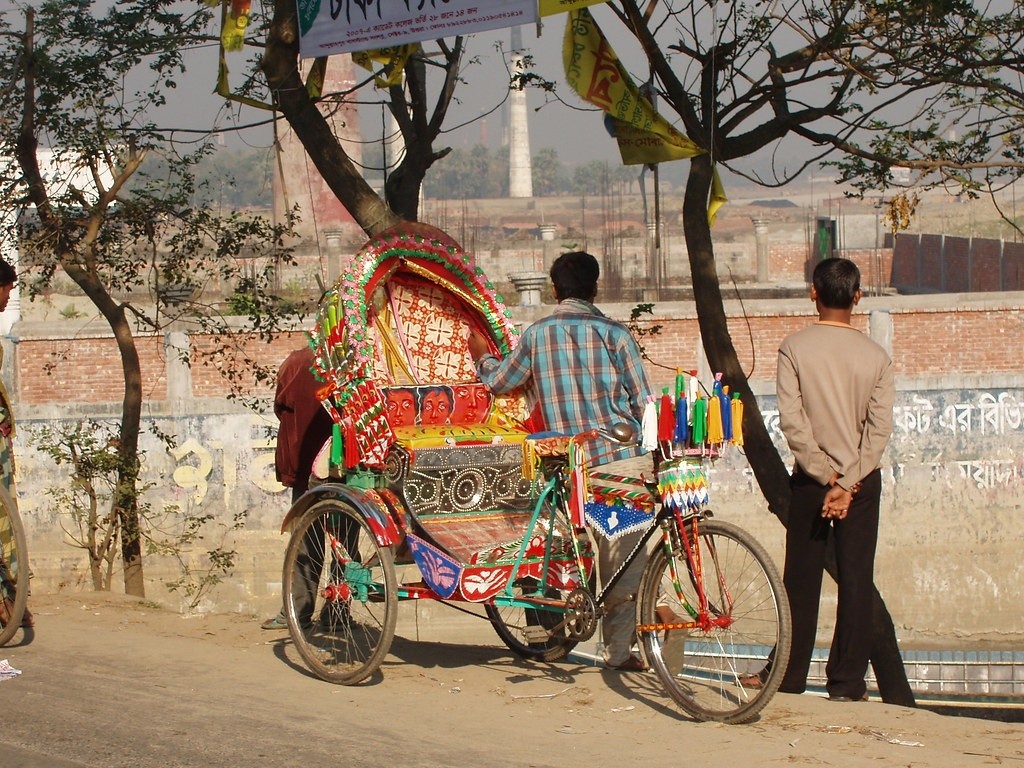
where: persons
[262,346,360,630]
[734,258,894,700]
[0,256,35,625]
[467,251,653,674]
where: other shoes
[0,597,34,627]
[320,621,356,632]
[829,691,868,701]
[604,654,645,671]
[734,670,780,689]
[661,618,687,677]
[262,613,313,629]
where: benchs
[376,382,548,517]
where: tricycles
[274,218,793,730]
